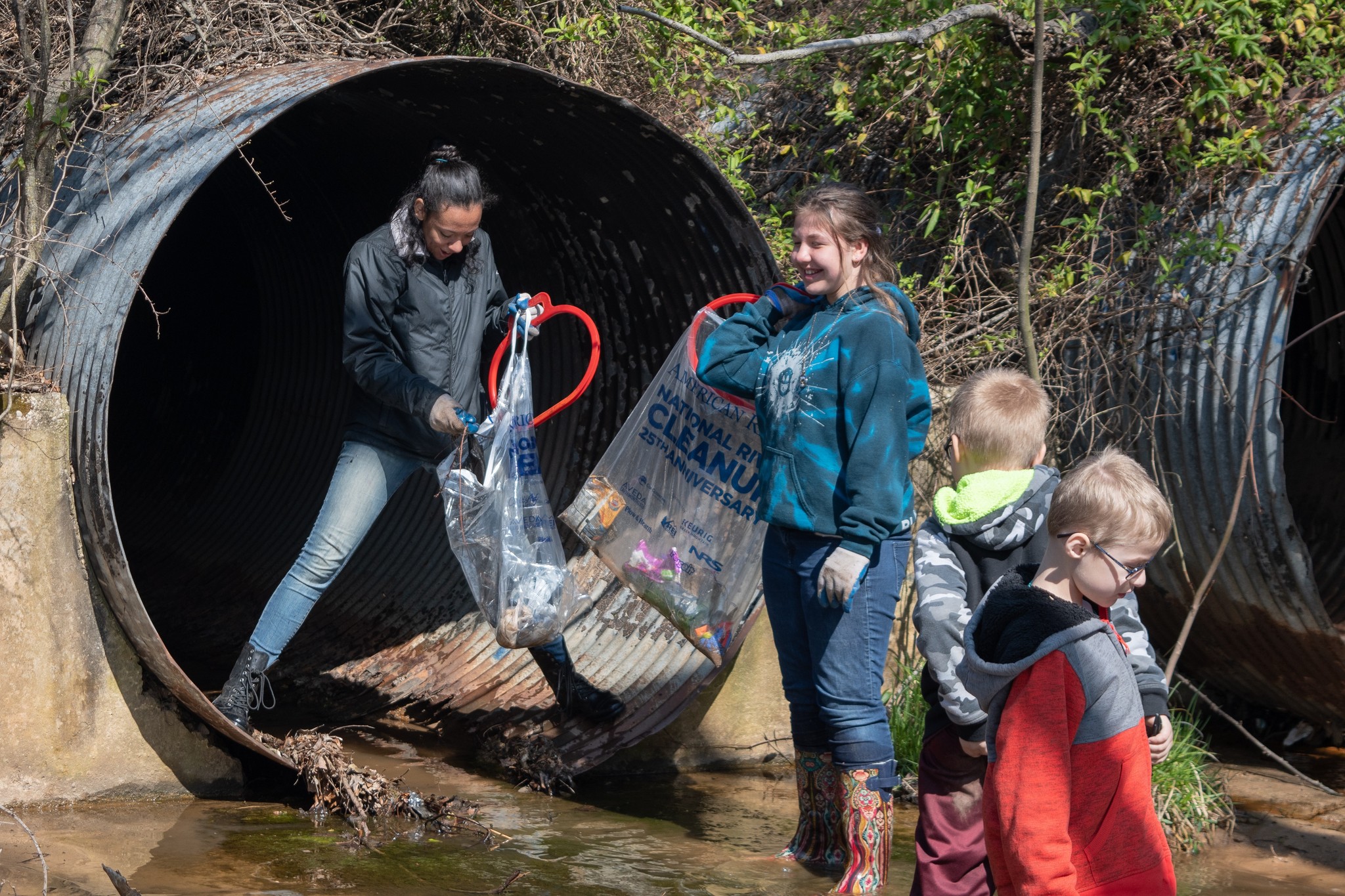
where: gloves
[429,393,480,435]
[508,291,545,340]
[816,546,873,614]
[767,279,825,318]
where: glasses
[1055,530,1154,581]
[943,432,958,460]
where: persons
[914,369,1175,896]
[694,184,932,896]
[215,145,625,733]
[958,444,1184,896]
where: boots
[532,634,625,722]
[212,640,281,731]
[828,757,900,896]
[770,748,846,865]
